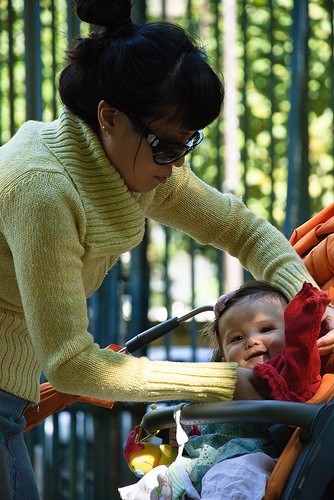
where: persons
[0,1,334,499]
[119,281,332,500]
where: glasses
[120,106,204,166]
